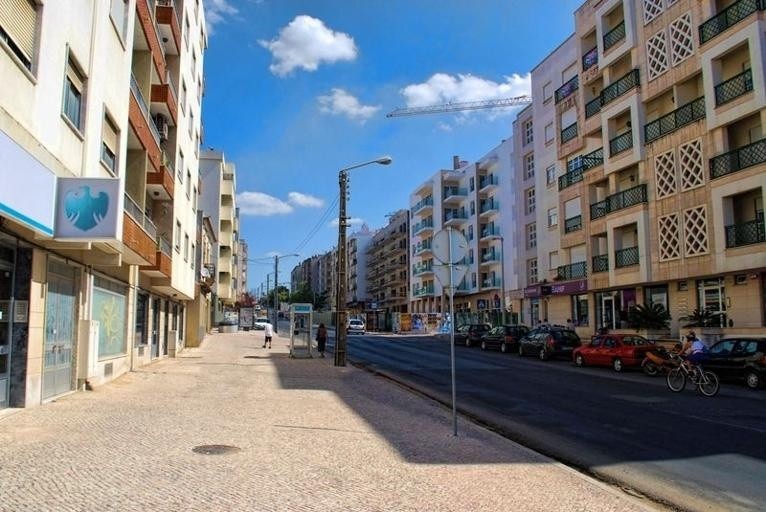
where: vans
[258,310,268,317]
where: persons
[543,319,550,326]
[566,317,576,332]
[262,318,274,349]
[536,319,543,330]
[315,323,329,357]
[678,329,710,384]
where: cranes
[386,95,531,118]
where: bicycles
[666,353,719,397]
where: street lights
[334,156,392,366]
[267,254,299,333]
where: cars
[255,305,261,312]
[347,319,365,335]
[254,316,267,329]
